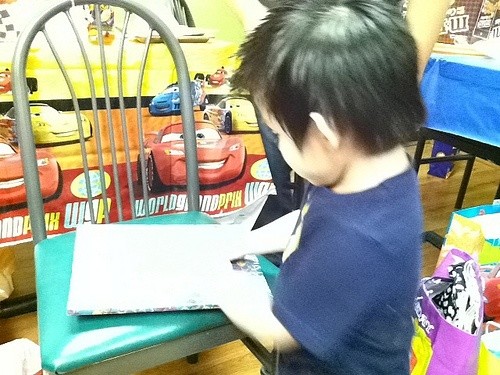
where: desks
[413,54,500,210]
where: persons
[172,0,451,375]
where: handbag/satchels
[478,321,500,375]
[408,248,484,375]
[435,199,500,271]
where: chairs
[11,0,280,375]
